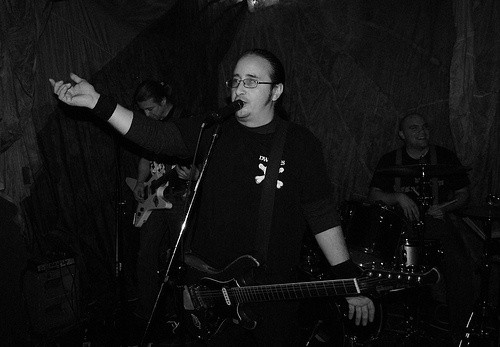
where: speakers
[24,266,81,336]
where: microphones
[203,100,243,127]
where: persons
[48,49,375,325]
[370,113,470,221]
[132,79,199,285]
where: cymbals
[454,205,500,221]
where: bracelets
[330,257,355,278]
[91,91,116,120]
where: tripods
[456,203,500,347]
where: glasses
[223,79,274,88]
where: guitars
[125,165,189,229]
[159,252,440,345]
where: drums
[366,201,415,266]
[341,199,367,235]
